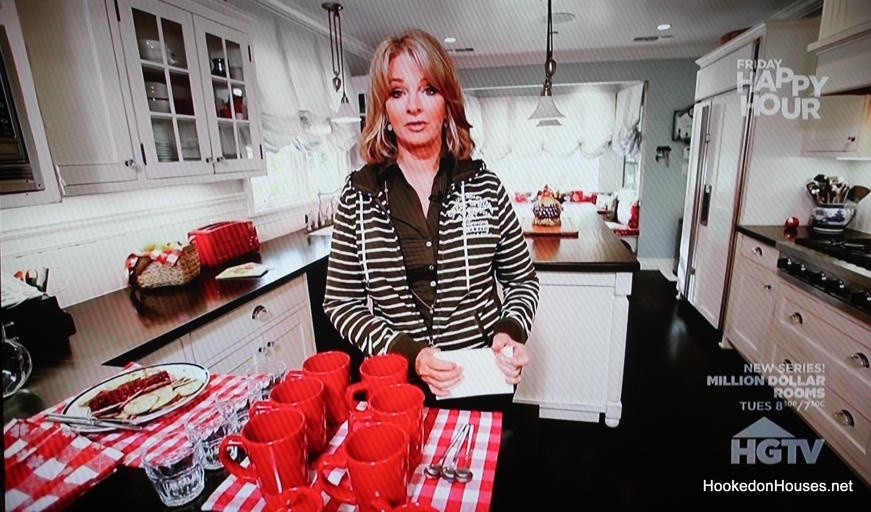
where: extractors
[808,2,871,98]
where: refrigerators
[670,16,806,334]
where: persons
[320,27,540,410]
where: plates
[155,139,174,160]
[55,361,212,436]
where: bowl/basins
[145,81,174,112]
[139,38,169,61]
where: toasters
[185,218,263,266]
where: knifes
[43,411,148,437]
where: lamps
[527,0,564,127]
[323,2,361,122]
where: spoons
[427,422,477,490]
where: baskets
[135,243,202,288]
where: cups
[213,57,231,75]
[142,349,426,511]
[218,91,246,119]
[809,204,858,233]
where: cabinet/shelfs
[717,234,775,381]
[105,0,269,187]
[138,274,317,386]
[9,0,139,197]
[758,279,868,499]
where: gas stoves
[775,236,871,326]
